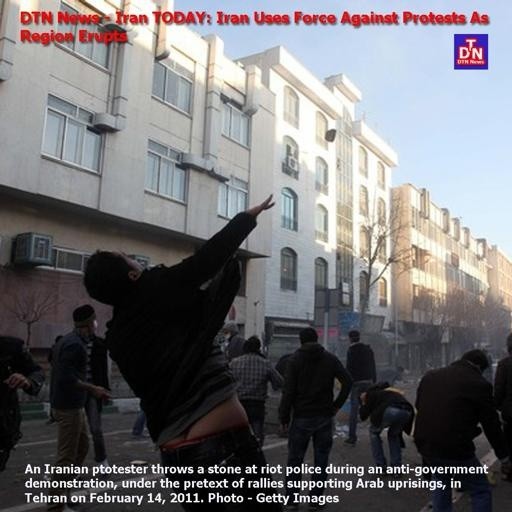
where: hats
[300,327,318,344]
[73,305,97,327]
[462,349,488,370]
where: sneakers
[48,459,110,511]
[376,464,402,474]
[344,438,356,443]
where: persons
[0,305,512,512]
[81,192,306,512]
